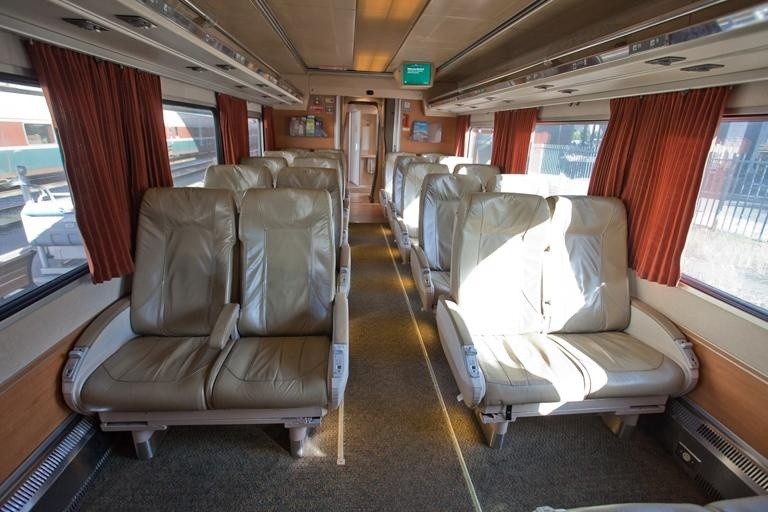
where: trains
[1,81,261,232]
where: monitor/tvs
[399,61,435,90]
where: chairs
[435,192,700,446]
[63,188,349,464]
[530,490,768,512]
[380,155,573,313]
[204,147,352,295]
